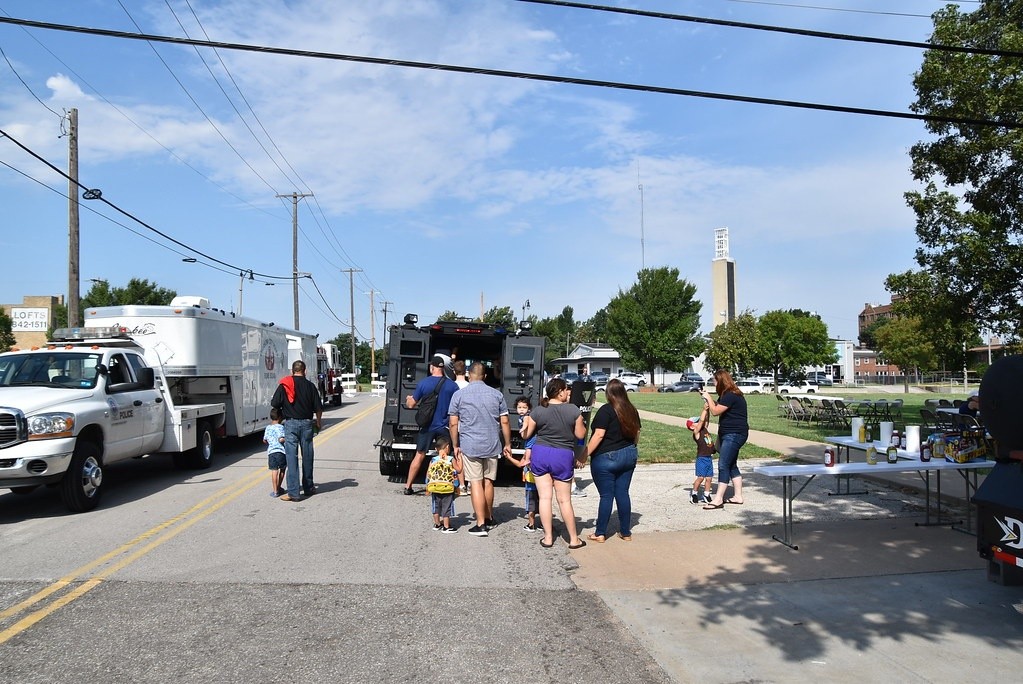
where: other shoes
[280,495,300,502]
[404,488,414,495]
[270,487,284,498]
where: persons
[263,408,287,498]
[513,396,533,434]
[575,379,641,543]
[434,340,502,495]
[686,402,716,504]
[271,360,322,501]
[447,363,512,536]
[784,371,802,388]
[700,371,749,510]
[526,378,586,550]
[425,437,463,534]
[578,367,593,382]
[959,388,979,418]
[404,356,460,496]
[504,434,547,532]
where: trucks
[0,295,320,516]
[373,312,548,477]
[316,344,343,408]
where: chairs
[919,398,979,436]
[776,394,905,433]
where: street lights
[522,299,531,321]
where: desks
[936,407,981,433]
[783,395,844,420]
[840,399,901,429]
[824,436,997,527]
[753,457,996,550]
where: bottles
[824,446,835,467]
[859,425,866,443]
[865,425,873,443]
[866,444,877,465]
[901,432,906,450]
[920,441,931,462]
[891,430,901,448]
[886,444,898,464]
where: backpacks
[426,452,454,494]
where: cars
[733,380,766,395]
[707,372,833,387]
[657,382,700,393]
[588,372,609,386]
[777,380,820,393]
[615,372,647,387]
[680,373,706,386]
[552,372,579,389]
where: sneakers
[537,524,544,531]
[441,527,457,533]
[432,523,444,531]
[702,490,713,503]
[689,489,698,505]
[484,516,498,530]
[523,523,536,533]
[458,485,472,496]
[467,524,488,537]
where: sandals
[587,534,605,543]
[617,532,631,541]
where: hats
[427,356,444,368]
[686,416,700,427]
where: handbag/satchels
[414,376,447,433]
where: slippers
[540,537,552,547]
[724,498,743,504]
[703,503,724,510]
[568,538,586,549]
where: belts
[285,418,299,420]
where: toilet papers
[880,421,893,446]
[905,425,921,453]
[852,418,864,441]
